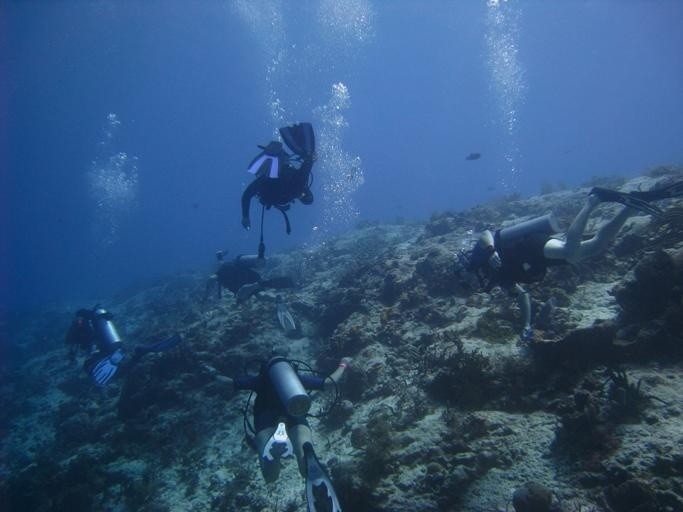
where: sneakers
[265,142,283,154]
[135,346,152,355]
[276,294,282,304]
[303,442,322,481]
[631,191,649,202]
[588,187,620,202]
[257,280,271,288]
[111,347,126,363]
[273,422,288,440]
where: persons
[203,248,283,303]
[202,356,353,483]
[241,152,313,232]
[69,309,153,367]
[453,193,640,340]
